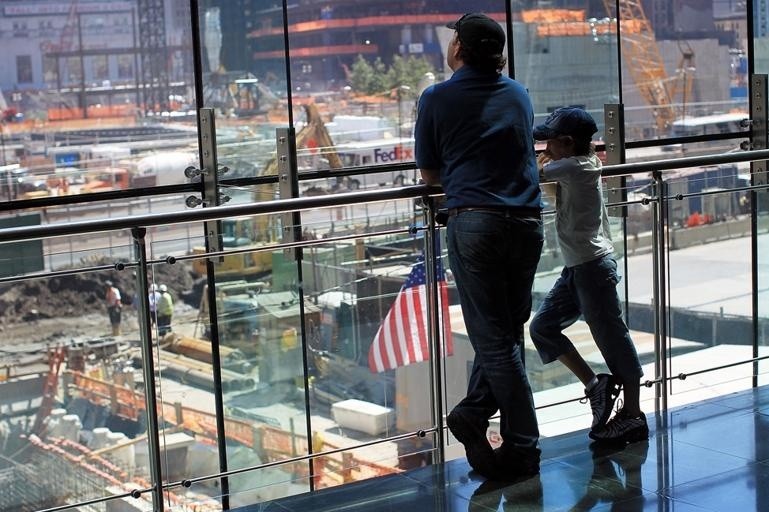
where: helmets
[104,280,168,292]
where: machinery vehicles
[265,96,333,127]
[182,98,350,300]
[81,152,201,194]
[202,278,265,335]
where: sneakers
[588,398,648,441]
[446,412,540,470]
[580,373,622,431]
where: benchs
[330,399,395,437]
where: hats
[533,107,597,141]
[445,12,505,54]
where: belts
[435,207,507,226]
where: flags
[367,226,454,374]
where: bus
[46,80,187,122]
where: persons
[529,105,650,444]
[415,12,543,475]
[567,440,646,512]
[467,472,544,512]
[102,279,173,337]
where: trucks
[0,163,47,198]
[661,114,748,156]
[310,264,459,367]
[336,138,416,189]
[627,165,750,221]
